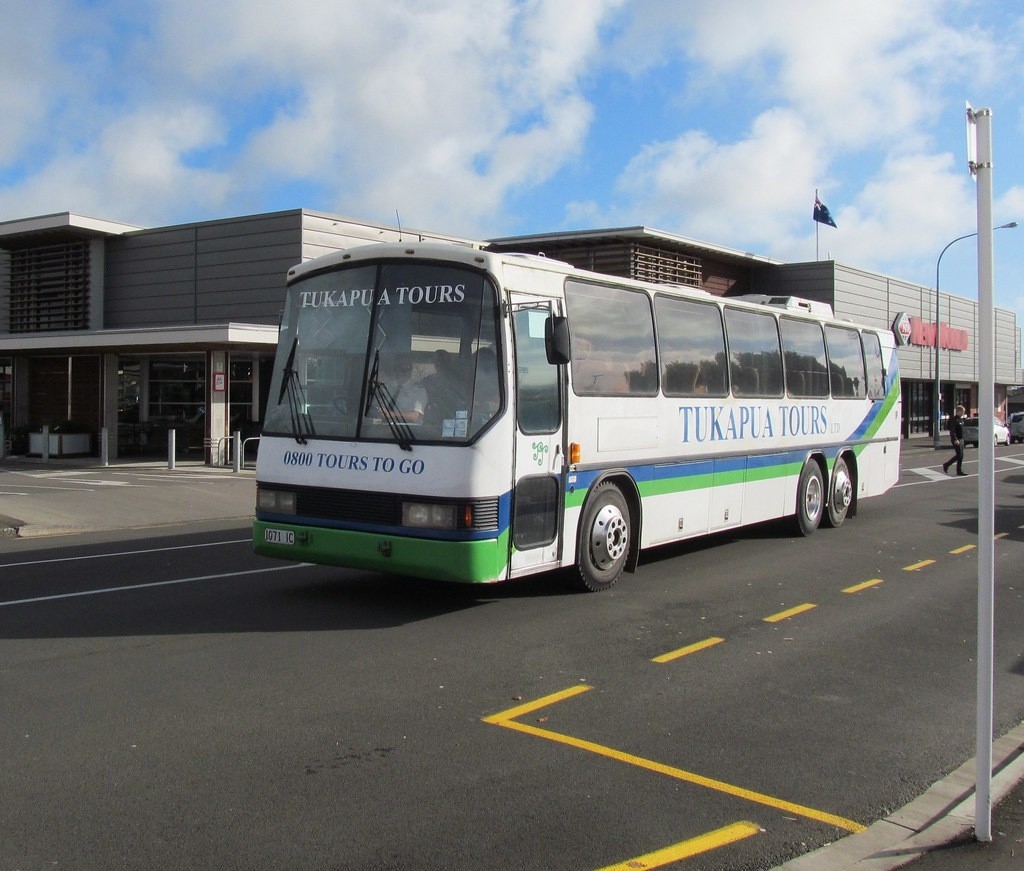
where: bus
[251,234,903,593]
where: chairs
[680,363,702,391]
[741,366,760,394]
[790,370,805,397]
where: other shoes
[943,464,948,473]
[957,472,968,476]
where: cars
[961,415,1012,447]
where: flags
[813,199,836,228]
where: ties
[387,385,401,410]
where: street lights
[933,222,1019,448]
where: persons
[367,354,427,424]
[469,347,501,403]
[943,406,968,475]
[422,348,461,403]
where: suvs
[1008,412,1024,442]
